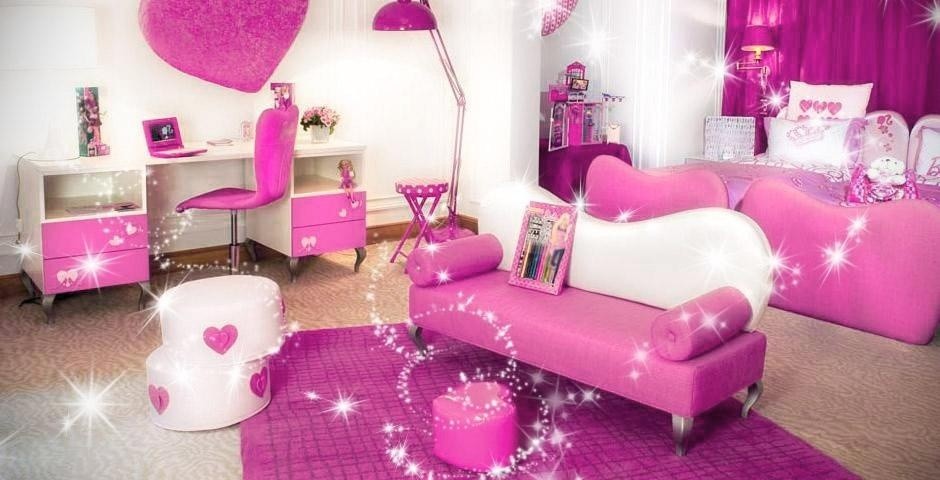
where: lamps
[372,0,467,240]
[735,24,774,77]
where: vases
[311,125,329,143]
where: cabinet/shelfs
[16,138,366,324]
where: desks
[540,138,632,204]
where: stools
[146,344,273,431]
[156,274,287,363]
[389,177,449,273]
[428,381,520,472]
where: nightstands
[685,153,715,164]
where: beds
[584,81,939,344]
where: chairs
[176,104,299,274]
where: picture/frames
[507,201,576,296]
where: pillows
[861,109,911,172]
[789,80,873,119]
[907,113,939,186]
[761,116,852,171]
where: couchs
[405,176,777,457]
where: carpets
[241,320,863,478]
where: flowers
[300,103,340,135]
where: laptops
[142,117,207,158]
